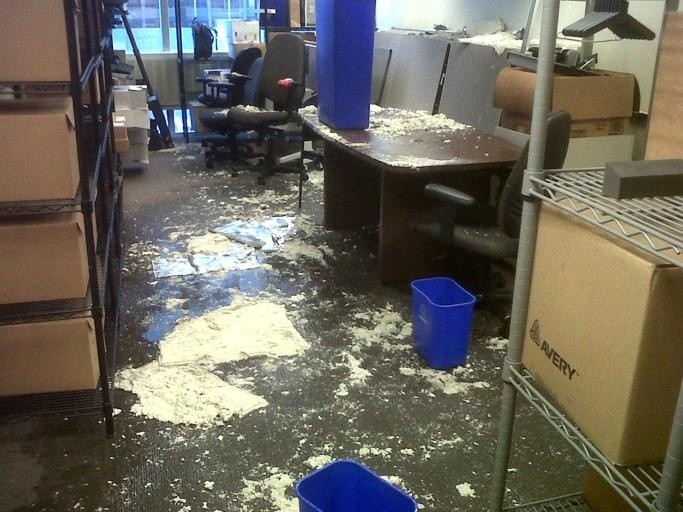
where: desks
[294,104,523,290]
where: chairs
[407,112,577,340]
[194,31,313,188]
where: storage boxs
[3,319,102,393]
[3,2,86,86]
[0,217,90,310]
[110,79,153,167]
[522,193,682,470]
[1,97,86,205]
[490,65,645,140]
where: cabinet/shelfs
[487,0,683,512]
[0,0,131,440]
